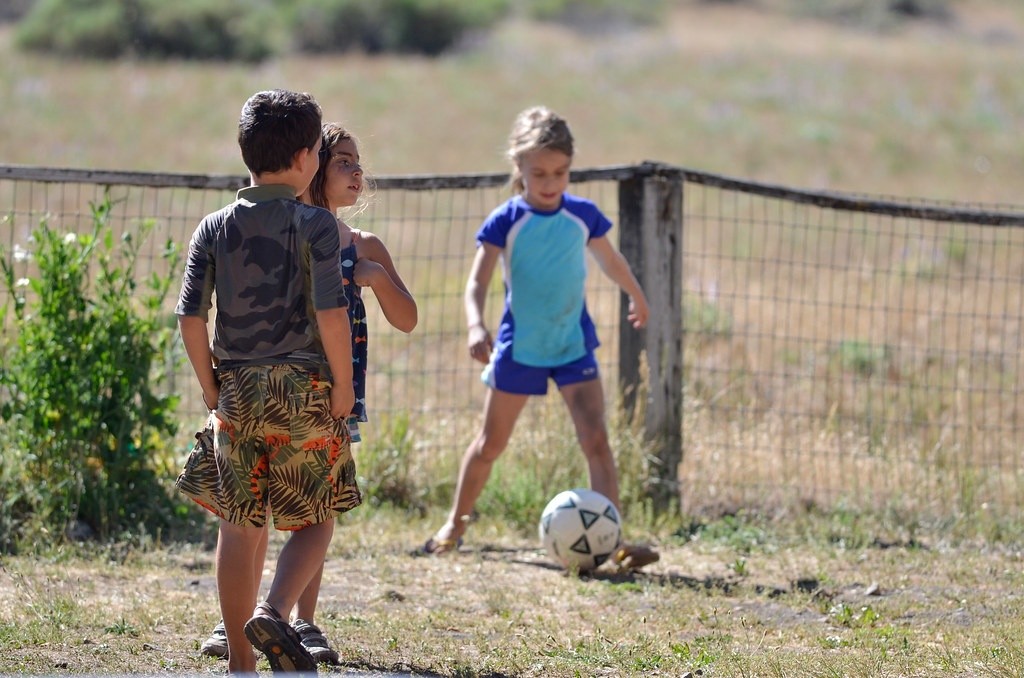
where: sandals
[245,602,319,678]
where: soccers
[538,486,624,571]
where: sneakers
[201,616,229,657]
[287,618,338,662]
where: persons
[424,106,660,568]
[198,121,418,662]
[174,90,362,678]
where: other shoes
[410,536,464,560]
[613,540,660,570]
